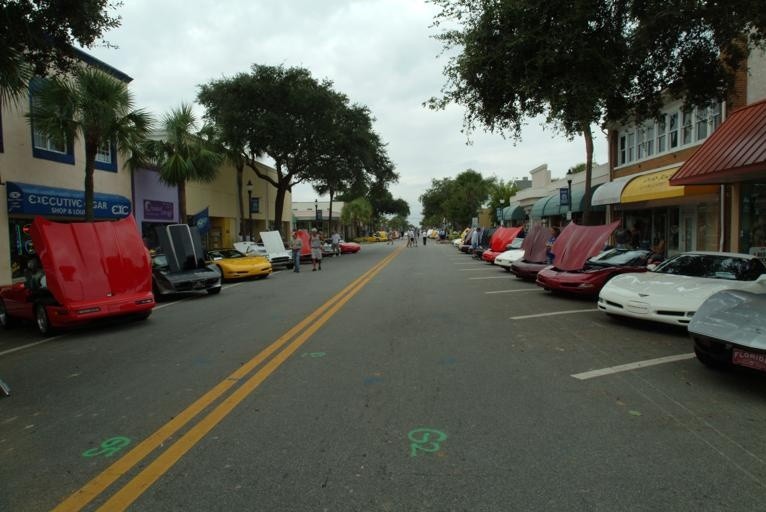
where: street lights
[565,168,575,221]
[314,198,319,227]
[499,197,506,227]
[245,177,255,242]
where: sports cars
[233,230,390,272]
[428,223,558,281]
[590,249,766,334]
[682,284,766,378]
[535,220,665,305]
[204,246,275,285]
[146,221,227,304]
[0,212,159,339]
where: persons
[545,226,561,266]
[284,227,341,273]
[470,228,480,258]
[387,229,427,248]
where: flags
[192,208,211,235]
